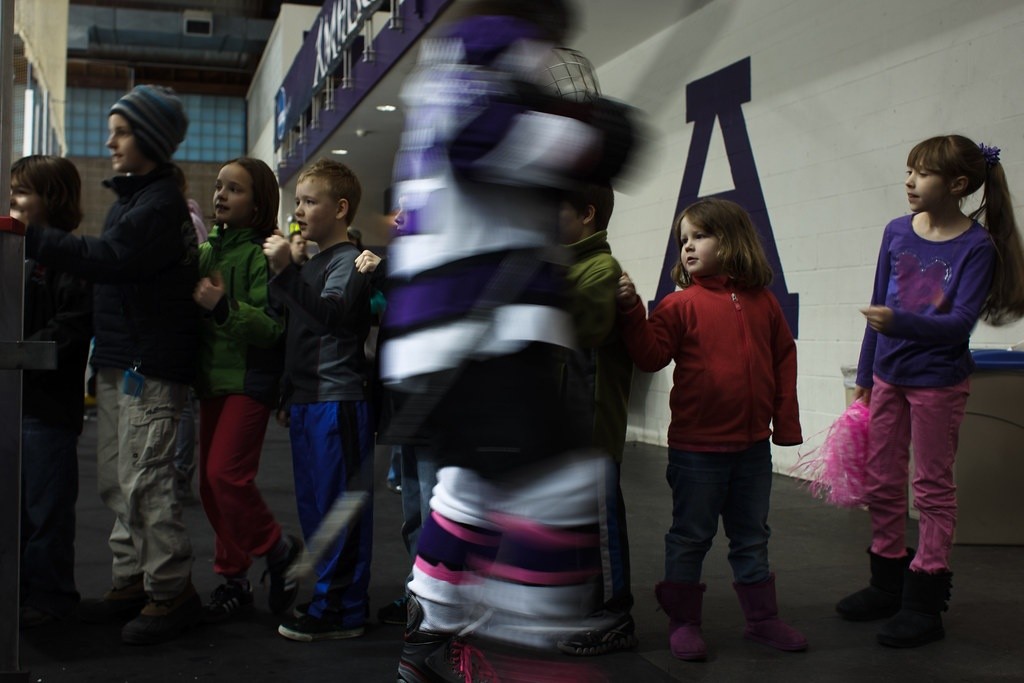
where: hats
[107,84,189,167]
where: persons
[287,230,311,263]
[353,0,637,683]
[262,158,372,639]
[9,84,199,646]
[553,182,634,655]
[196,158,305,622]
[11,155,94,628]
[162,163,207,244]
[620,198,808,661]
[837,135,1024,650]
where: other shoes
[20,589,83,625]
[387,480,401,492]
[78,587,148,623]
[122,583,206,647]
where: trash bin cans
[841,365,858,409]
[908,348,1024,548]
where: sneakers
[557,610,641,657]
[293,601,312,616]
[377,596,408,626]
[264,534,306,618]
[274,611,367,641]
[201,577,259,628]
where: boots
[732,572,810,654]
[834,545,914,623]
[875,568,953,647]
[653,575,711,662]
